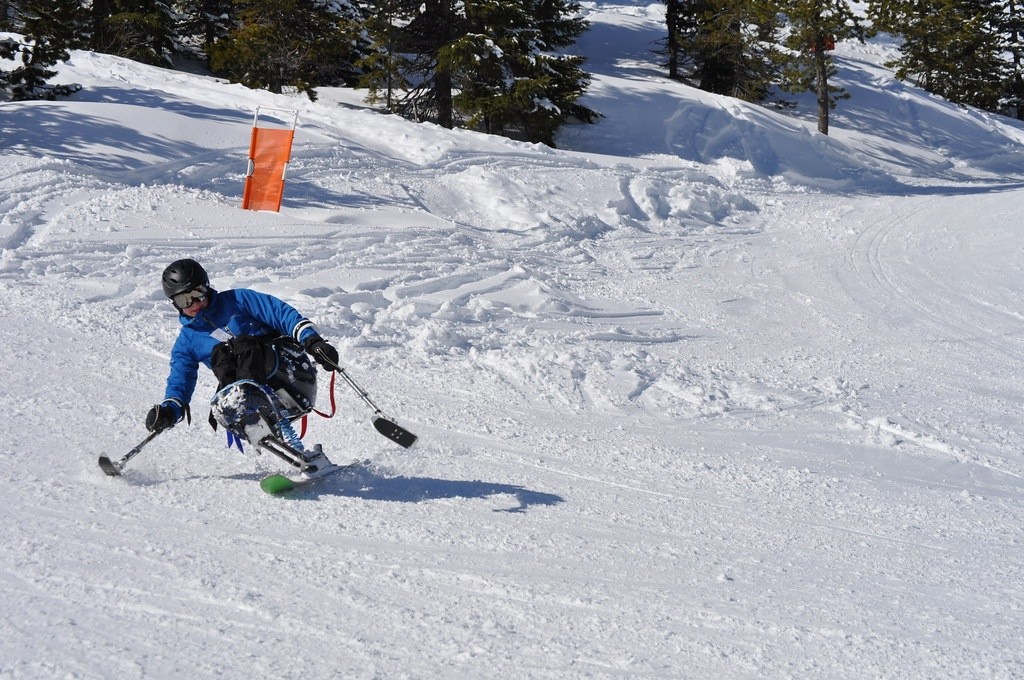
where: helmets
[161,259,208,299]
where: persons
[145,258,340,432]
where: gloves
[305,335,339,372]
[146,406,176,432]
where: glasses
[173,284,209,309]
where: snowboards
[259,457,363,495]
